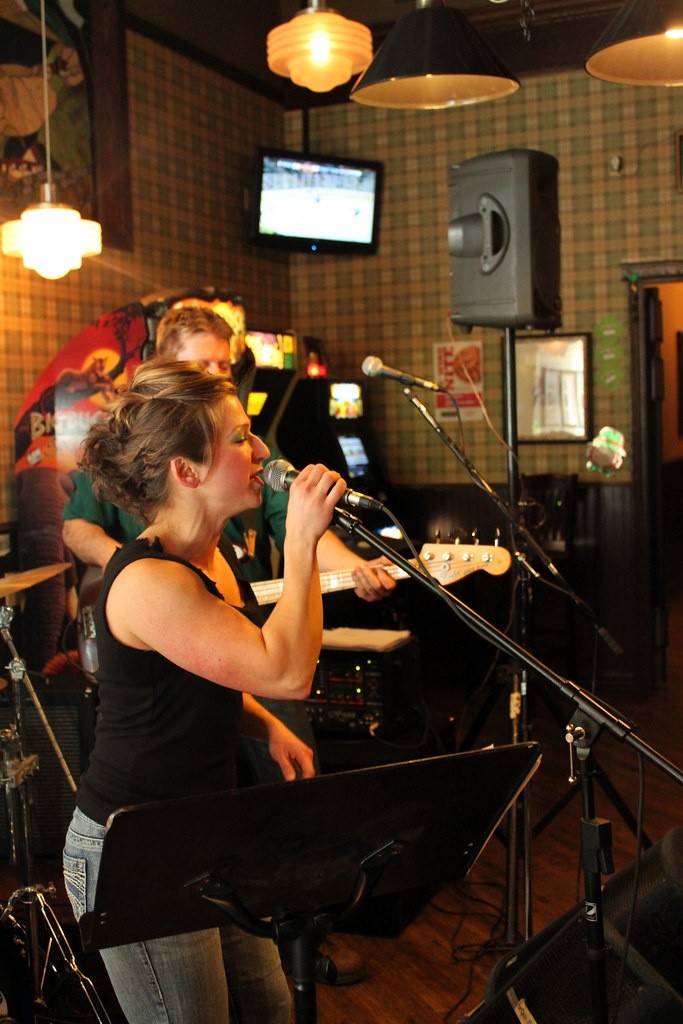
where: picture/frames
[504,331,592,444]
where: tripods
[0,605,112,1024]
[457,328,655,850]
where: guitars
[251,526,511,608]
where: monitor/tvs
[251,145,384,256]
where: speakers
[446,147,562,335]
[0,677,100,856]
[458,824,683,1024]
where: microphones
[361,356,447,394]
[261,459,385,515]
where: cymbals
[1,560,71,598]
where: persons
[62,353,347,1024]
[62,304,396,981]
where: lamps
[586,0,682,86]
[0,0,102,281]
[351,0,521,108]
[265,0,376,93]
